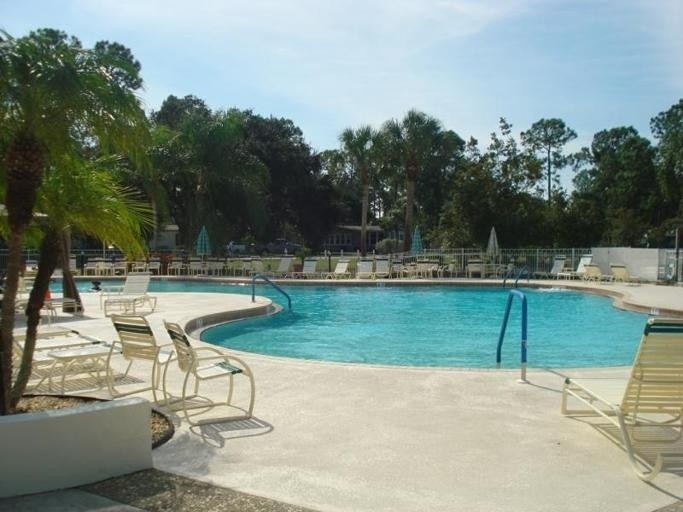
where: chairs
[13,273,255,427]
[83,253,640,285]
[561,317,683,482]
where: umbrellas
[0,205,53,227]
[61,225,84,312]
[411,224,424,254]
[485,226,500,257]
[196,225,212,256]
